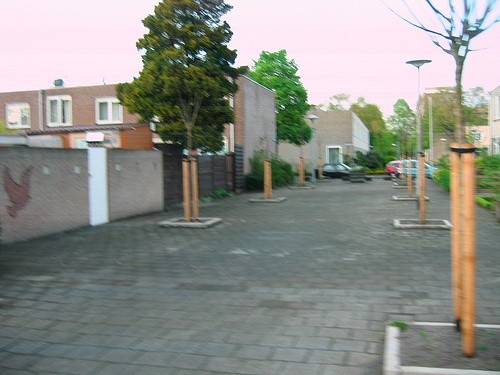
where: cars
[403,160,439,179]
[313,162,371,180]
[384,160,404,178]
[304,114,320,182]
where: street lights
[406,59,432,210]
